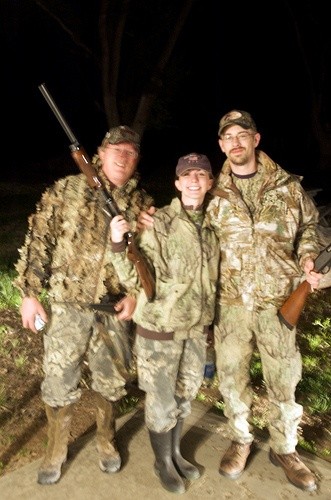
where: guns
[276,245,331,332]
[37,82,155,304]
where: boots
[172,417,199,480]
[147,426,185,494]
[37,404,74,483]
[92,390,122,473]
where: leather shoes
[219,440,255,479]
[269,447,315,491]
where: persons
[135,111,331,490]
[110,155,221,494]
[12,125,153,484]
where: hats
[102,126,141,152]
[218,110,252,136]
[175,153,211,176]
